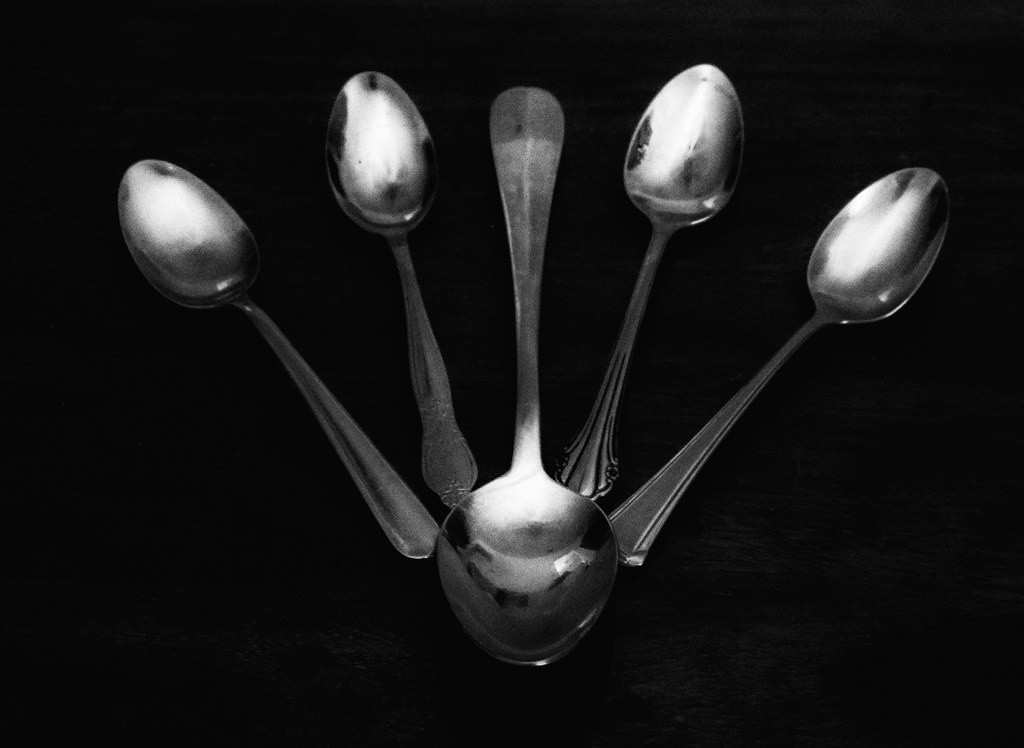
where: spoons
[607,167,952,566]
[556,63,745,494]
[322,70,481,509]
[435,88,617,667]
[119,159,440,559]
